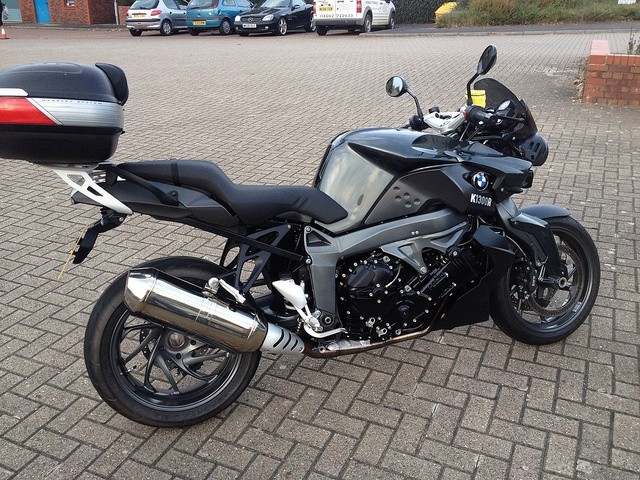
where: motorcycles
[0,45,600,428]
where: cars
[233,0,316,35]
[125,0,188,36]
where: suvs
[185,0,253,35]
[313,0,395,34]
